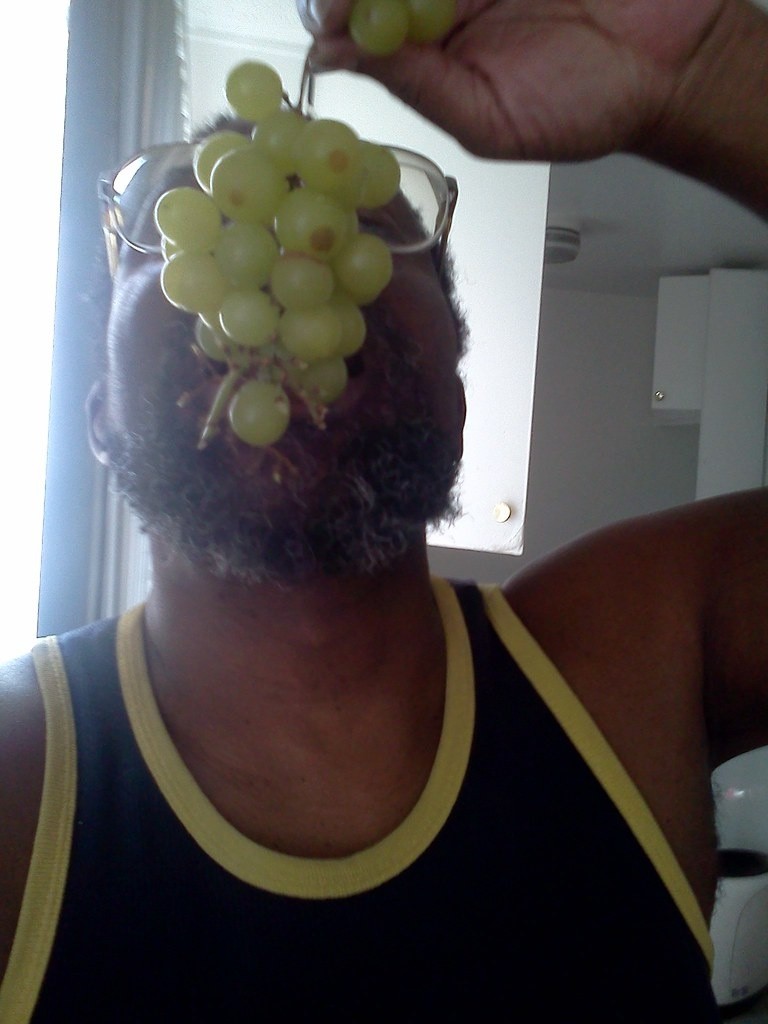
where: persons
[0,0,768,1024]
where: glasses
[97,139,458,279]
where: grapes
[150,1,459,448]
